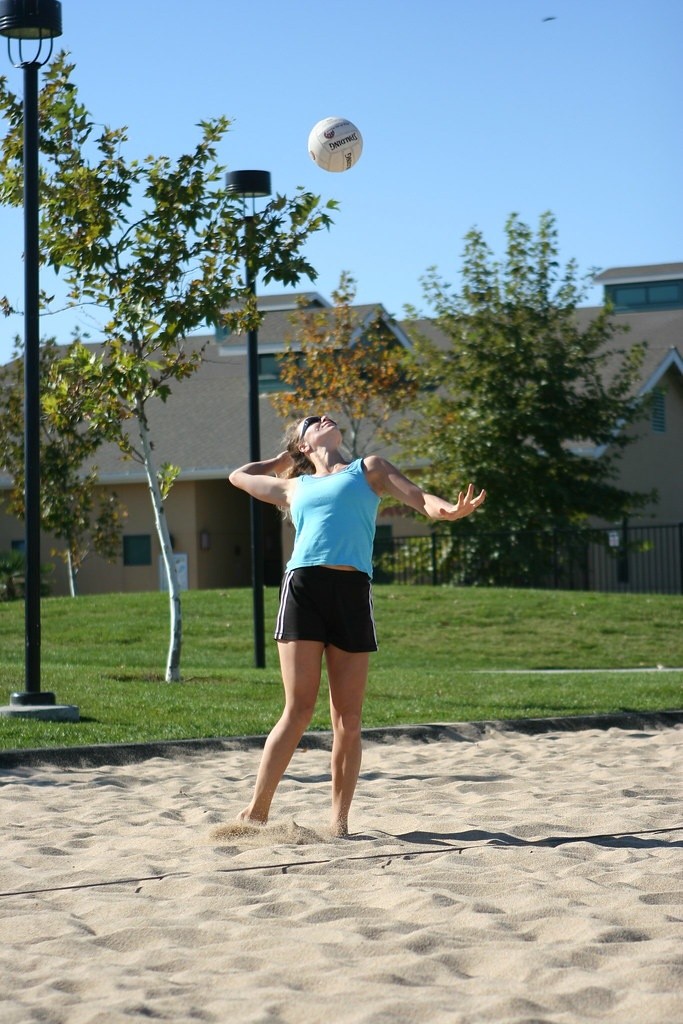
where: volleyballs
[306,115,365,174]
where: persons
[228,414,487,835]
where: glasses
[300,417,336,439]
